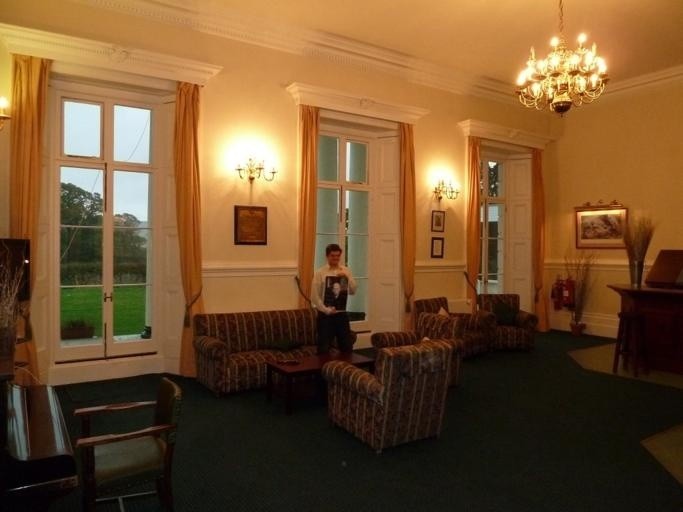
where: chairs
[320,339,453,454]
[477,293,539,351]
[72,375,183,512]
[413,296,491,356]
[369,311,465,386]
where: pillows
[438,307,450,318]
[491,301,517,326]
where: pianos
[1,327,79,511]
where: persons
[325,282,348,310]
[310,243,358,355]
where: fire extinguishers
[551,274,575,311]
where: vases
[628,259,645,287]
[568,322,585,338]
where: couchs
[190,307,357,392]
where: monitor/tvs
[0,238,30,302]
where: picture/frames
[574,205,629,249]
[231,204,268,246]
[430,236,443,258]
[430,210,445,233]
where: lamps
[433,179,460,203]
[513,1,610,116]
[0,96,13,131]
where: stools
[611,309,650,378]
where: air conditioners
[236,158,277,186]
